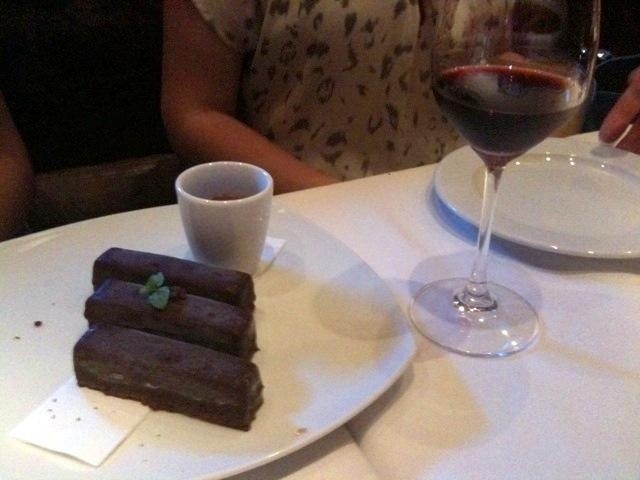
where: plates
[1,199,420,480]
[432,135,640,259]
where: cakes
[85,280,259,362]
[92,248,255,311]
[73,328,264,434]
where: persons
[162,0,583,194]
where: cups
[175,160,276,277]
[409,0,603,364]
